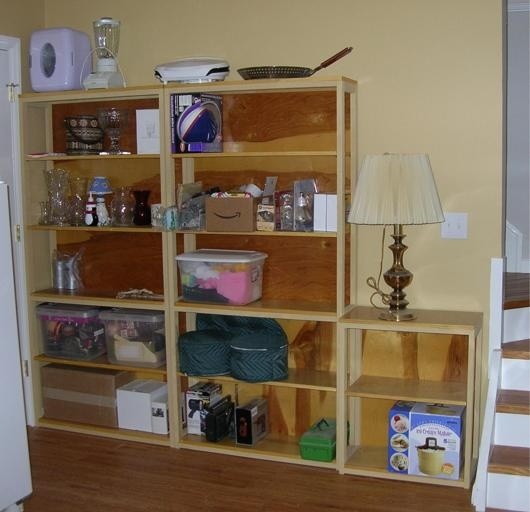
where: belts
[95,107,131,155]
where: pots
[237,45,354,80]
[416,436,446,476]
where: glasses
[346,152,446,322]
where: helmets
[390,413,409,471]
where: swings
[80,14,127,90]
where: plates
[335,303,485,490]
[18,75,359,470]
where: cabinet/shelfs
[35,169,151,227]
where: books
[298,415,350,461]
[387,400,466,481]
[34,301,169,435]
[177,313,291,384]
[173,247,269,307]
[203,190,274,233]
